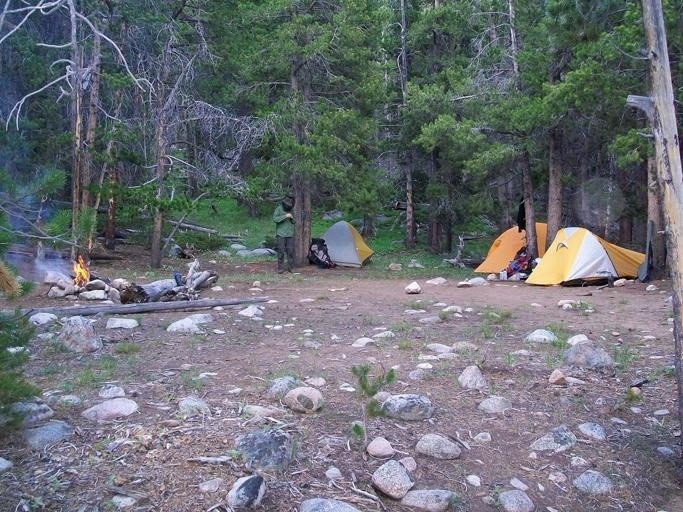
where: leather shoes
[278,269,284,274]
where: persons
[272,194,297,274]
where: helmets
[282,192,295,212]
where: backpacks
[308,238,331,269]
[501,246,533,280]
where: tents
[319,221,376,269]
[473,221,547,274]
[524,227,645,287]
